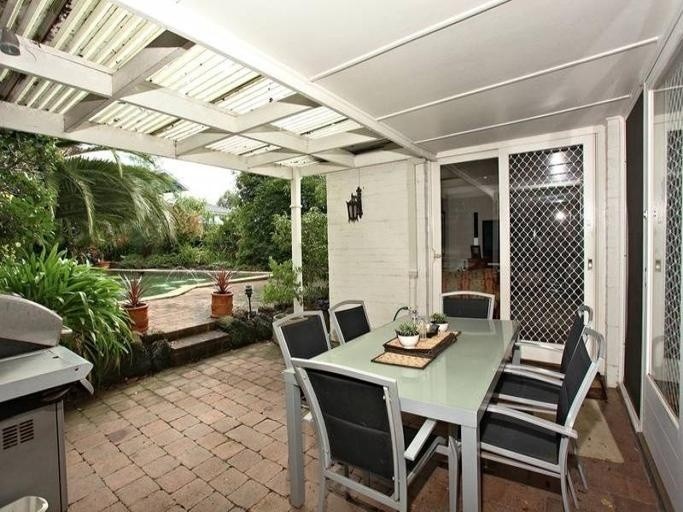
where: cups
[396,334,420,348]
[429,321,448,332]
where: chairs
[441,290,496,319]
[447,327,604,512]
[289,356,446,512]
[328,299,372,345]
[273,311,330,411]
[496,305,594,491]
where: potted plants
[122,270,151,334]
[428,313,448,332]
[395,320,421,348]
[206,261,240,318]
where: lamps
[346,187,363,224]
